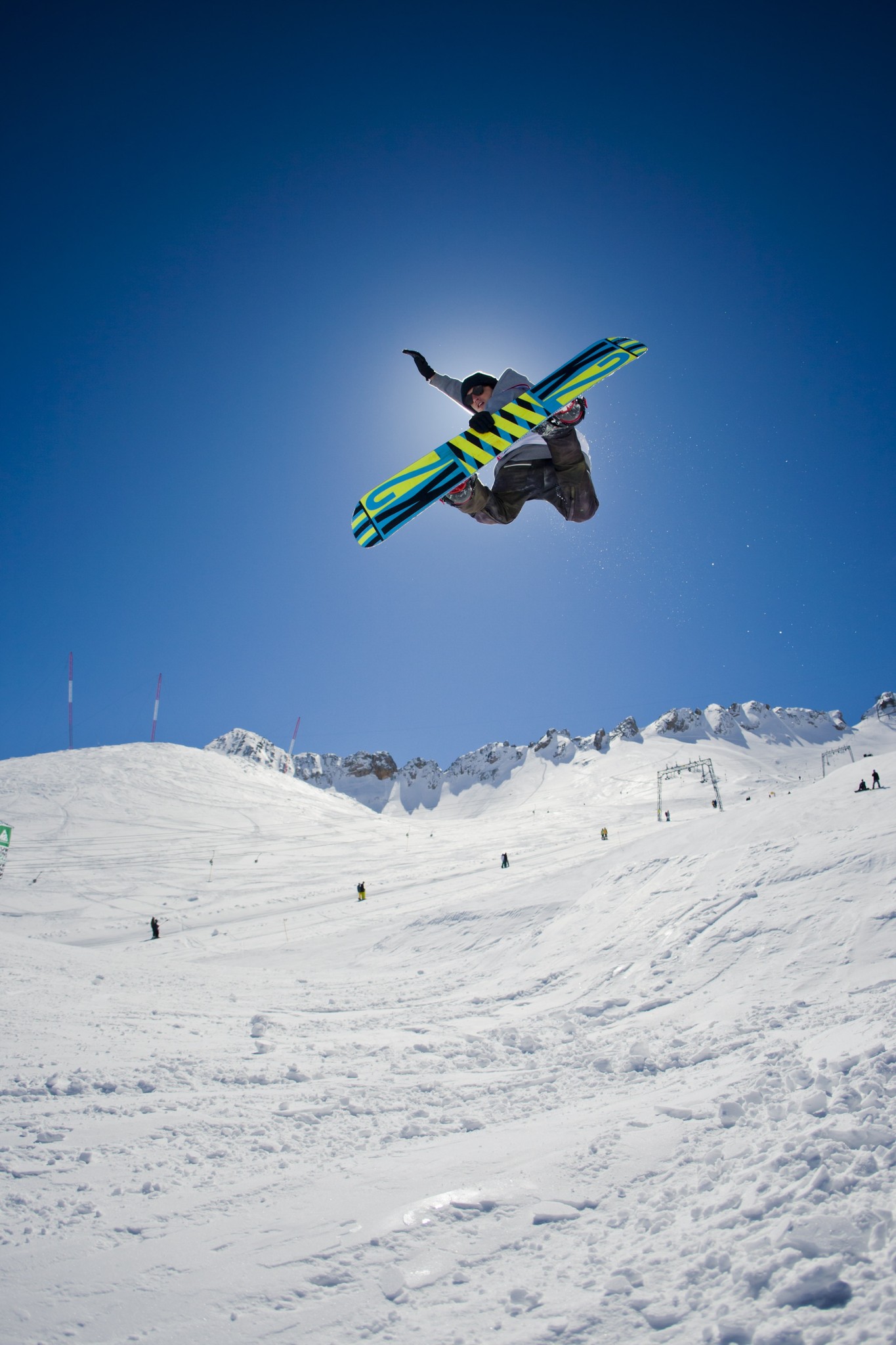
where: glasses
[463,385,484,405]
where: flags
[0,824,11,882]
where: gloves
[402,349,436,381]
[468,411,496,434]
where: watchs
[799,776,801,780]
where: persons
[604,827,608,839]
[857,779,867,790]
[154,919,160,939]
[667,810,670,821]
[400,349,599,526]
[601,829,605,840]
[712,800,715,808]
[361,881,367,900]
[746,796,750,800]
[788,791,791,795]
[502,853,506,868]
[357,882,363,900]
[504,852,510,868]
[714,799,717,808]
[872,769,881,790]
[151,916,156,939]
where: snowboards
[351,336,649,547]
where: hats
[461,372,499,414]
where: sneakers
[438,476,478,506]
[548,396,589,428]
